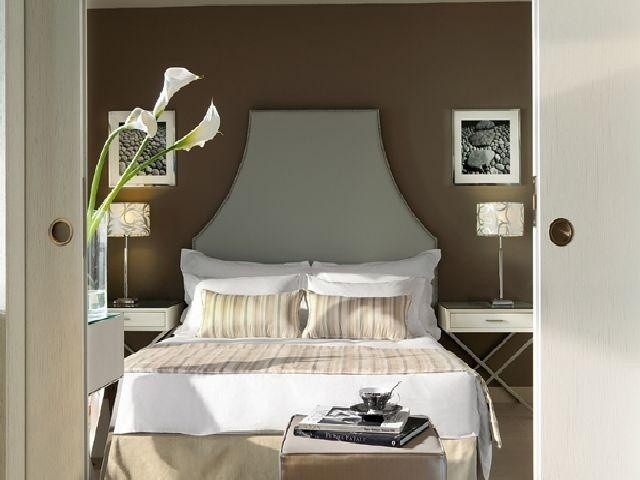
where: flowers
[84,64,221,247]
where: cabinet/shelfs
[85,314,130,403]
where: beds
[97,109,504,479]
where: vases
[84,210,111,321]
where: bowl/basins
[358,393,393,410]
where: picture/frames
[106,109,178,187]
[450,106,523,187]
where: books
[299,403,410,433]
[293,417,428,447]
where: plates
[348,403,404,417]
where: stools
[278,411,448,480]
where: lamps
[105,203,153,306]
[475,201,527,308]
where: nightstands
[104,296,183,353]
[438,299,531,413]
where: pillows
[197,290,302,341]
[303,290,412,344]
[183,274,302,338]
[178,245,310,323]
[305,275,426,340]
[310,248,443,339]
